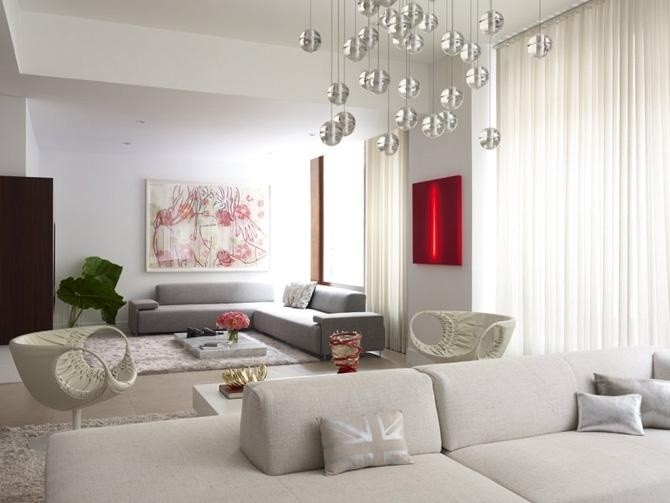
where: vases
[226,328,238,342]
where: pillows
[652,351,670,379]
[315,408,413,476]
[593,373,670,430]
[576,390,645,436]
[283,281,318,310]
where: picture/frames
[146,177,271,271]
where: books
[219,384,244,398]
[200,343,224,349]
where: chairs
[411,310,516,360]
[9,325,137,433]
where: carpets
[0,409,202,503]
[83,329,322,375]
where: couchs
[129,282,385,363]
[47,348,669,503]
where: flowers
[216,311,250,330]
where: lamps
[299,0,551,154]
